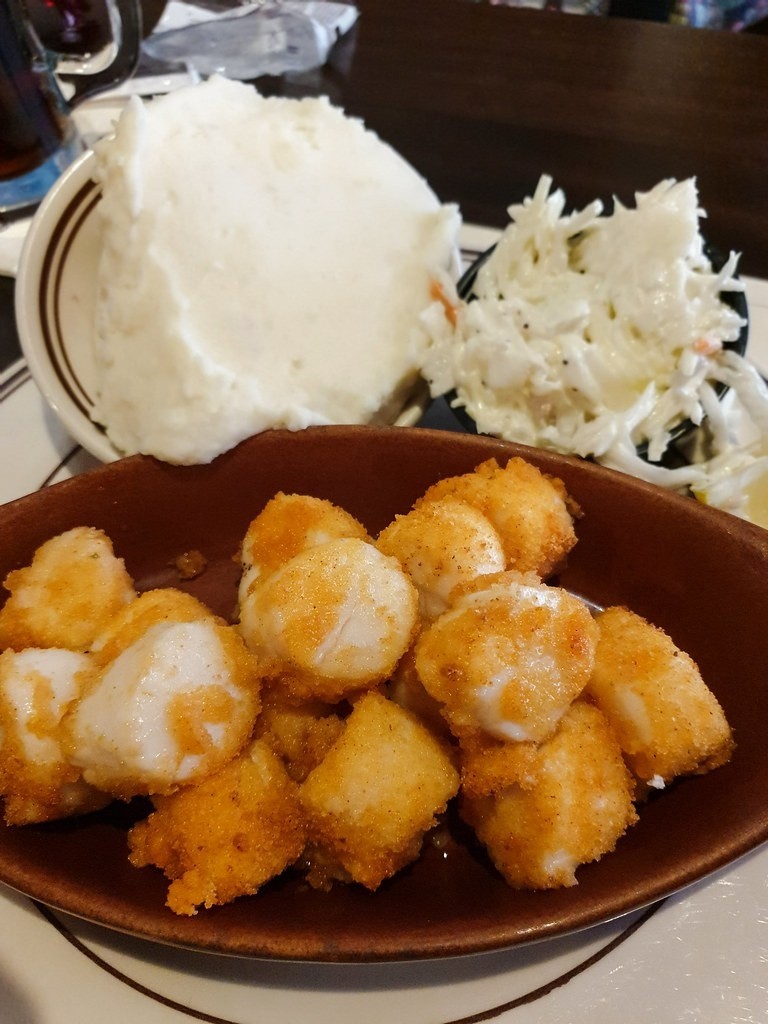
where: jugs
[0,1,143,213]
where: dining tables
[0,0,768,1024]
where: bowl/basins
[442,213,748,461]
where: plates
[15,132,462,465]
[0,424,767,965]
